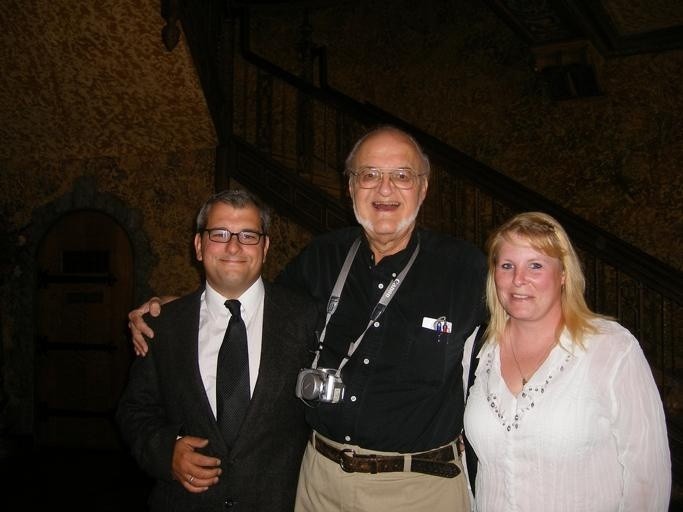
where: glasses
[195,226,267,247]
[351,169,426,190]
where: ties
[215,299,251,450]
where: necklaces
[509,329,558,388]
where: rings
[188,477,194,483]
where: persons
[118,187,317,512]
[126,120,476,512]
[462,210,670,512]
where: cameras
[295,366,346,408]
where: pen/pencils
[436,321,449,347]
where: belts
[306,438,461,479]
